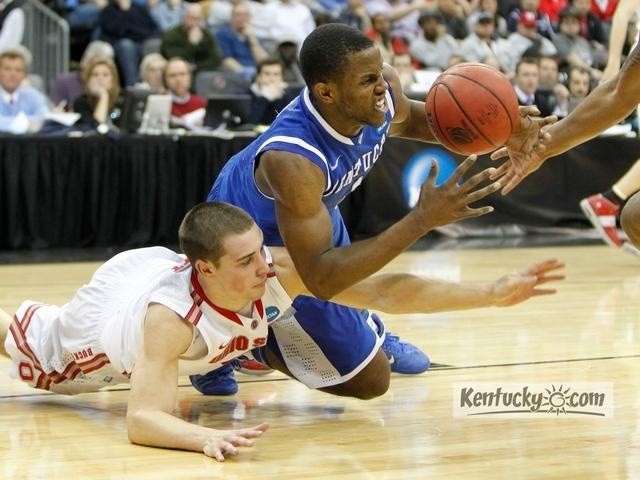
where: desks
[2,123,640,254]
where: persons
[0,200,564,463]
[579,0,640,249]
[190,22,557,402]
[490,43,640,251]
[0,0,638,136]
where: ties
[119,87,159,134]
[203,95,252,129]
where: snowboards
[425,62,518,156]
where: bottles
[478,11,495,23]
[275,35,299,50]
[520,9,538,28]
[418,8,442,27]
[559,6,580,21]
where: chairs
[234,355,275,378]
[579,192,623,251]
[382,331,431,376]
[189,359,239,397]
